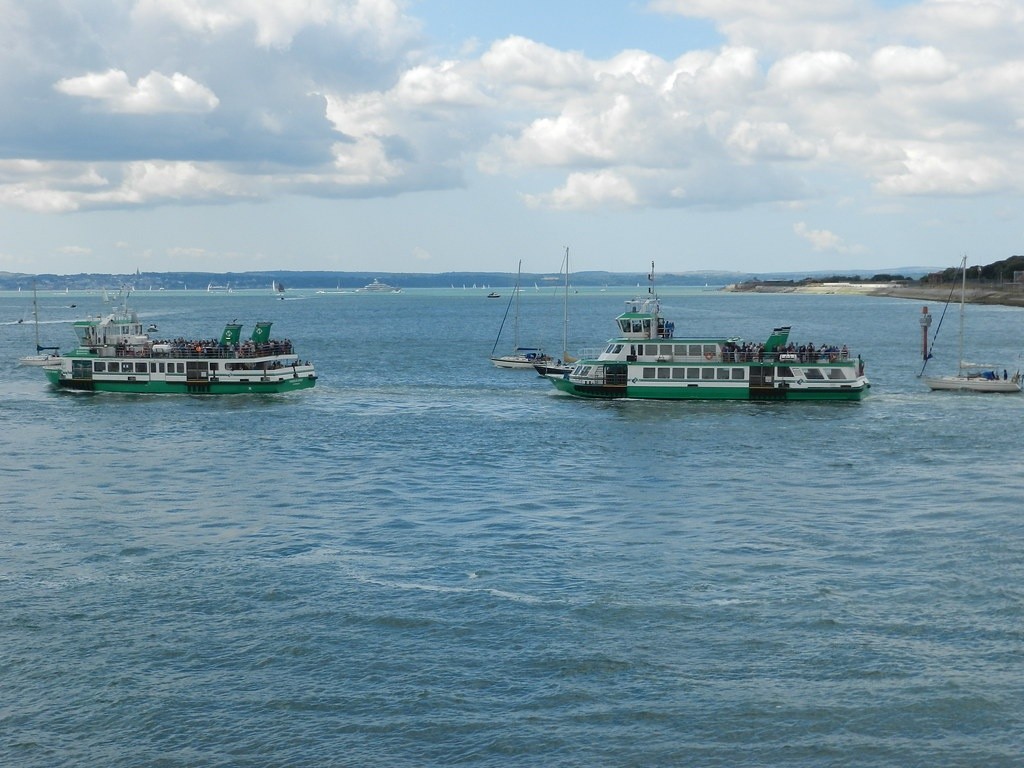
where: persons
[144,342,149,355]
[627,320,630,327]
[722,341,840,363]
[995,375,999,380]
[54,350,60,357]
[130,343,133,351]
[119,342,126,350]
[537,354,540,359]
[841,343,848,357]
[229,342,240,358]
[292,359,311,366]
[1003,369,1007,380]
[665,321,675,337]
[152,336,218,358]
[241,337,292,358]
[272,361,281,369]
[558,359,561,364]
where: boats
[355,279,402,293]
[42,282,318,393]
[487,291,501,297]
[544,261,871,401]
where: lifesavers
[194,345,203,353]
[832,353,838,359]
[705,351,713,360]
[238,349,244,356]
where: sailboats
[916,251,1024,393]
[489,259,554,369]
[19,280,62,366]
[272,281,285,292]
[532,245,579,378]
